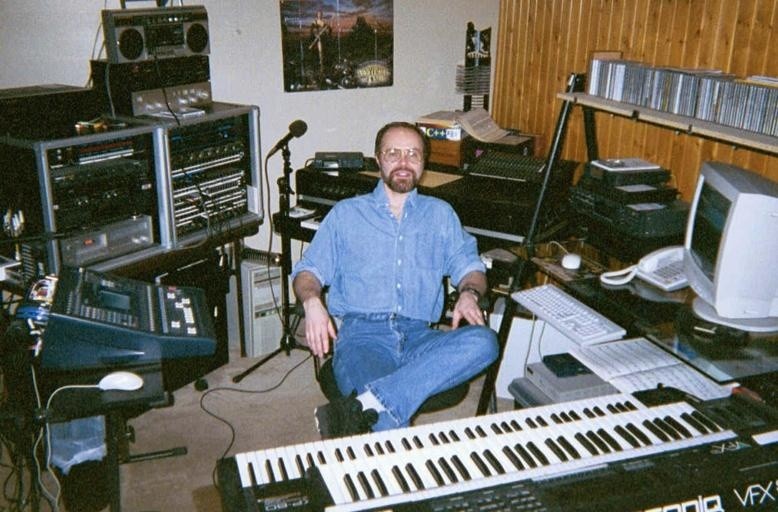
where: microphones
[266,119,308,157]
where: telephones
[635,245,688,292]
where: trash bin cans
[46,415,109,512]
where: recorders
[101,4,211,61]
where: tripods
[234,163,313,380]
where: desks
[43,360,193,512]
[496,230,776,384]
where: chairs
[306,279,468,440]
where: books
[567,336,732,402]
[415,110,510,143]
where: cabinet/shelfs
[102,220,262,393]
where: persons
[291,121,501,437]
[310,9,332,72]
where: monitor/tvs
[683,160,778,332]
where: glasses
[378,150,424,165]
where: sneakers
[313,389,379,440]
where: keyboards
[510,282,627,347]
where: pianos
[217,384,778,511]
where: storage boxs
[416,110,478,169]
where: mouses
[97,370,145,392]
[562,253,581,269]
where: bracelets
[462,287,480,299]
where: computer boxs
[225,260,283,359]
[489,296,568,399]
